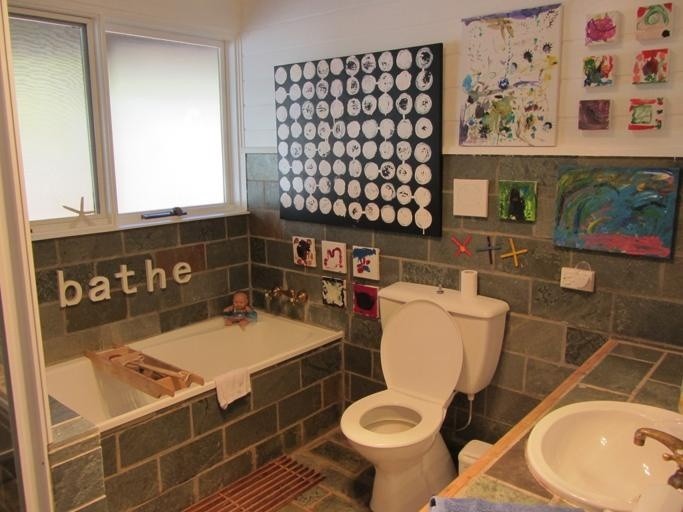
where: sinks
[525,399,683,510]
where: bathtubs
[43,310,344,431]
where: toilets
[338,281,511,511]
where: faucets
[632,427,683,469]
[271,288,294,298]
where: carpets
[180,455,327,512]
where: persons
[221,291,257,327]
